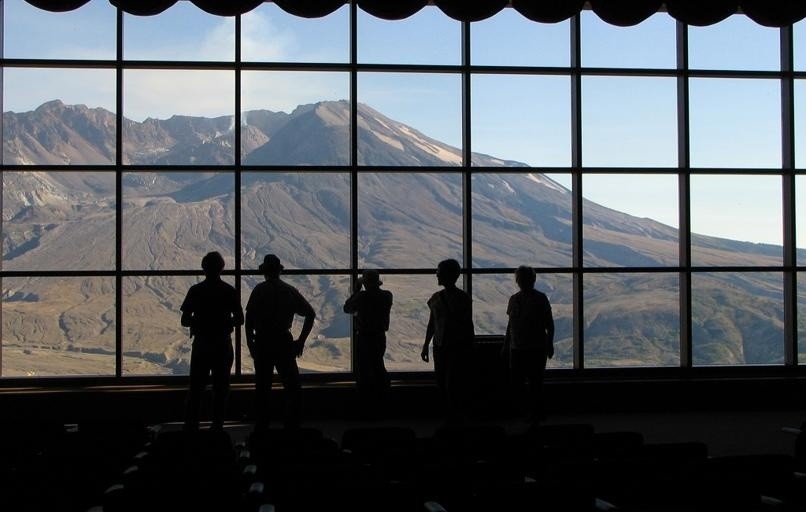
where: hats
[359,270,383,287]
[258,254,284,274]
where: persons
[240,254,316,427]
[422,258,474,421]
[343,271,394,423]
[501,263,555,425]
[181,251,245,432]
